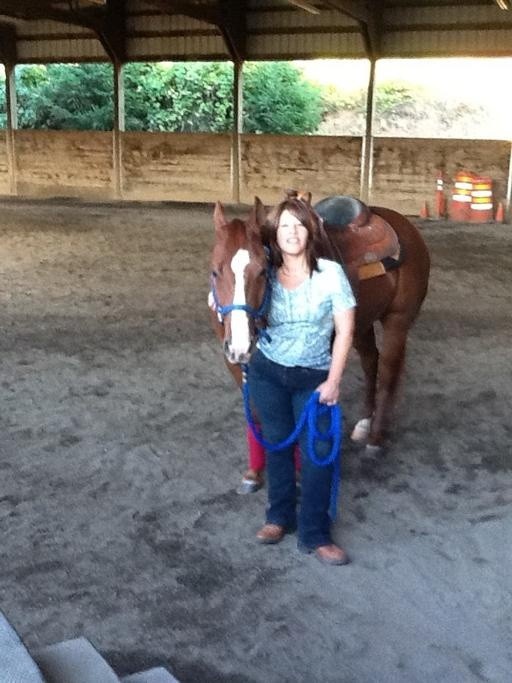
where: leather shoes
[256,523,284,544]
[297,538,348,565]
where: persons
[207,197,359,566]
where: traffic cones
[494,202,508,224]
[418,200,430,219]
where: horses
[206,195,433,496]
